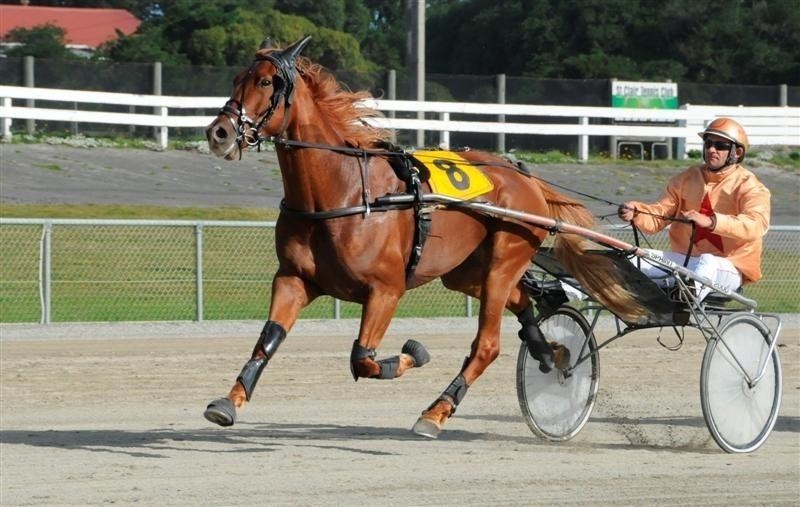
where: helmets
[698,118,748,163]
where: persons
[518,118,772,326]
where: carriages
[203,36,784,455]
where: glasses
[705,140,730,150]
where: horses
[203,34,650,441]
